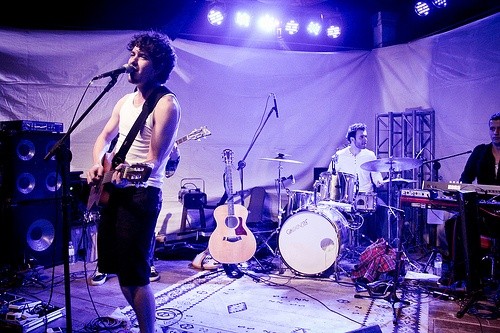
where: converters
[433,292,449,300]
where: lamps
[413,0,453,16]
[207,0,343,39]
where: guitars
[175,125,211,146]
[208,148,257,265]
[86,151,153,212]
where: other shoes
[149,265,160,281]
[90,266,108,286]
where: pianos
[421,180,500,297]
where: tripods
[353,203,411,324]
[256,162,284,256]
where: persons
[91,86,180,285]
[438,114,500,286]
[87,31,182,333]
[328,124,385,205]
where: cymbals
[360,157,424,173]
[258,157,305,164]
[381,178,420,184]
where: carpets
[112,268,429,333]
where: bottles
[68,241,75,263]
[434,253,442,276]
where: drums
[278,204,350,275]
[316,172,358,205]
[351,191,377,213]
[286,190,316,215]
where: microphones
[94,63,135,81]
[290,174,295,184]
[424,148,440,170]
[272,94,279,118]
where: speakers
[0,130,70,201]
[1,201,65,272]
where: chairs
[242,186,269,273]
